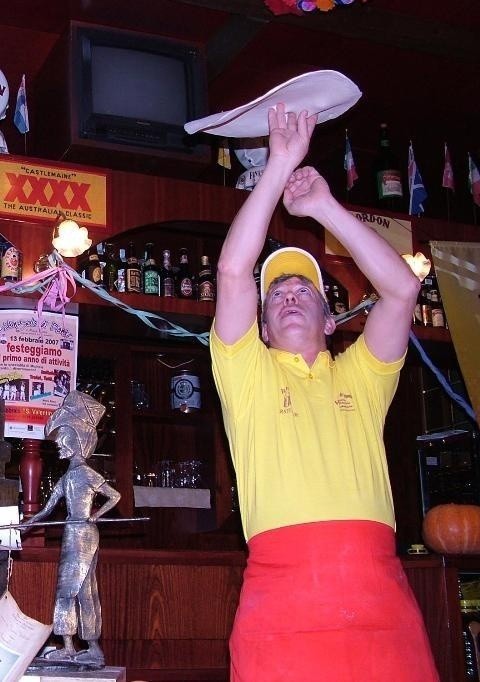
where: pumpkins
[422,503,479,552]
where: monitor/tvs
[36,20,212,182]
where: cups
[408,545,426,554]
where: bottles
[376,122,405,210]
[461,600,480,682]
[411,270,447,329]
[170,353,203,412]
[40,476,65,509]
[84,244,214,301]
[324,280,347,315]
[134,458,201,489]
[1,242,20,284]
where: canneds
[169,369,201,410]
[458,572,479,615]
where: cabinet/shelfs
[5,336,449,550]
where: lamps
[360,253,431,316]
[33,213,92,273]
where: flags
[13,78,30,135]
[343,132,360,194]
[216,136,232,173]
[467,155,480,205]
[405,142,428,217]
[441,146,457,194]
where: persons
[10,391,121,668]
[207,101,442,681]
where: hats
[257,243,332,314]
